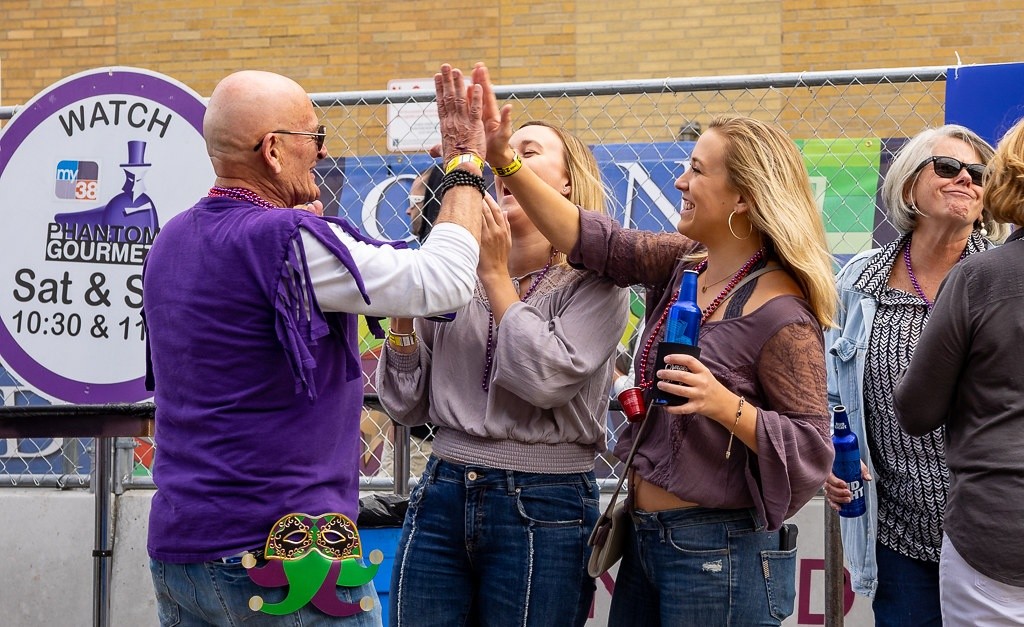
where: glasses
[254,124,327,152]
[914,156,990,188]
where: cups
[617,387,646,423]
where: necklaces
[618,245,770,423]
[208,186,279,211]
[482,249,566,391]
[904,237,965,308]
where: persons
[893,120,1024,627]
[360,169,433,515]
[821,124,1013,627]
[142,63,485,627]
[467,65,838,627]
[613,313,645,398]
[379,167,439,477]
[376,121,630,627]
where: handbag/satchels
[586,499,627,579]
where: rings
[303,201,313,205]
[822,488,827,495]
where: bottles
[652,269,702,407]
[424,311,458,322]
[828,405,867,519]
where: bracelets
[388,328,416,346]
[440,154,486,199]
[491,150,523,176]
[726,396,745,459]
